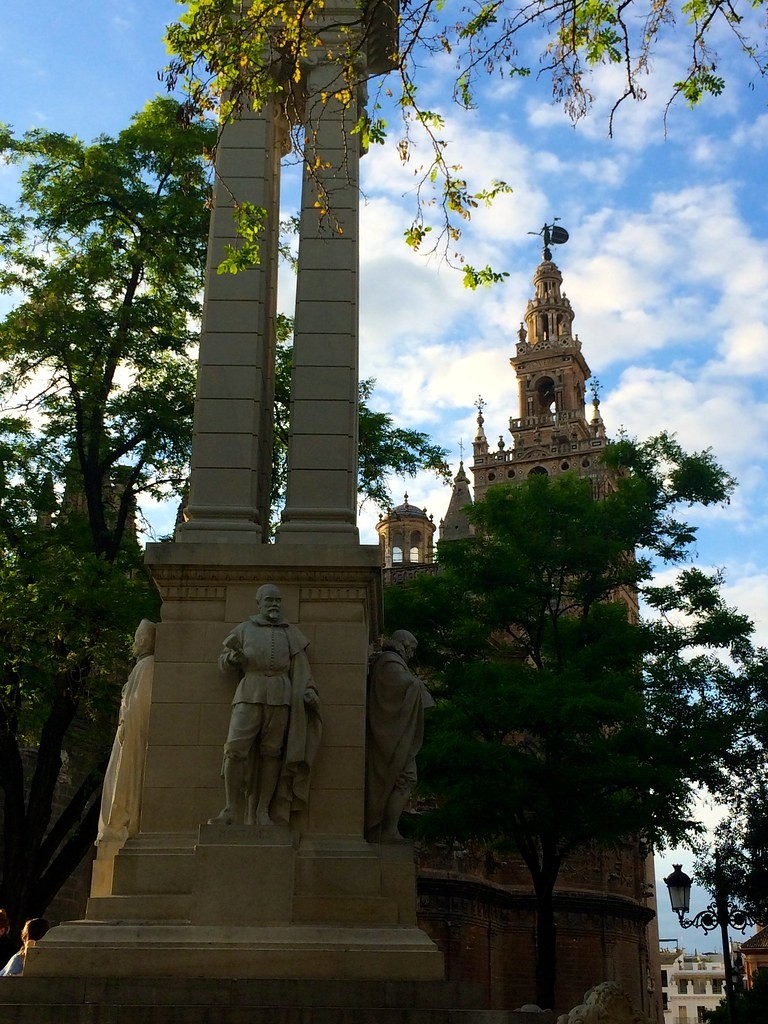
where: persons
[94,618,157,836]
[540,222,555,248]
[0,906,48,977]
[206,583,323,826]
[366,629,435,842]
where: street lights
[664,848,760,1023]
